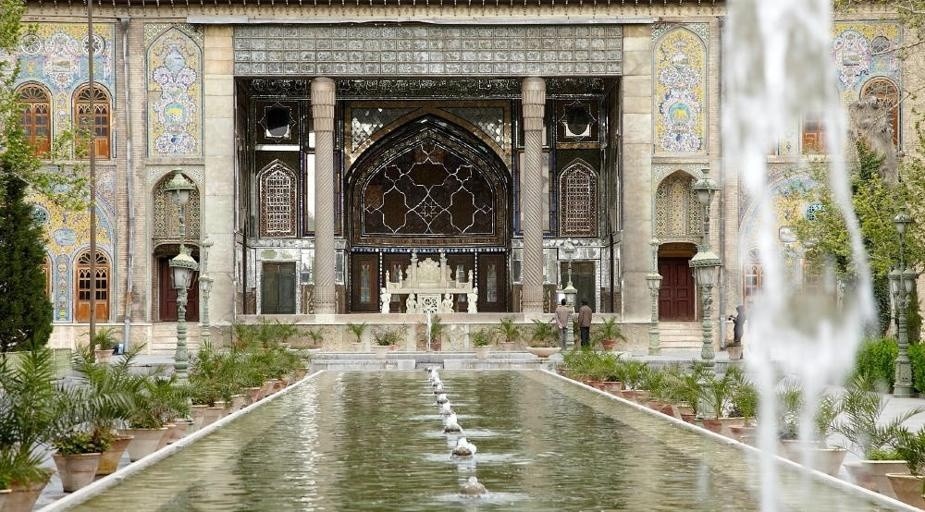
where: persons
[727,305,746,346]
[577,299,593,350]
[554,298,572,351]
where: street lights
[886,203,917,401]
[559,235,578,353]
[688,151,727,423]
[644,230,666,357]
[160,160,203,391]
[198,232,214,345]
